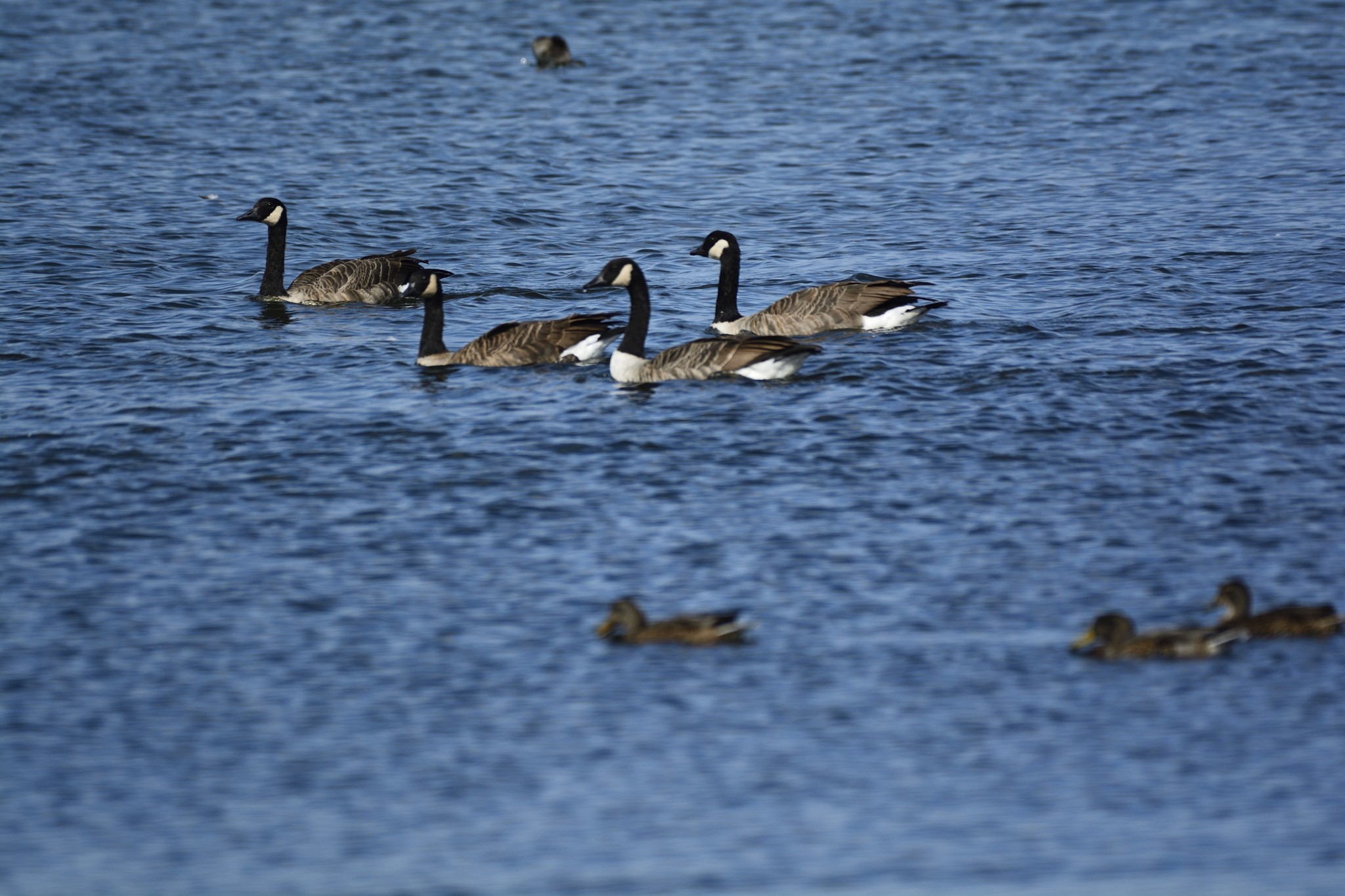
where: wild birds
[531,34,575,68]
[690,230,949,336]
[235,197,456,308]
[396,271,631,368]
[581,258,827,384]
[599,599,756,645]
[1063,575,1345,663]
